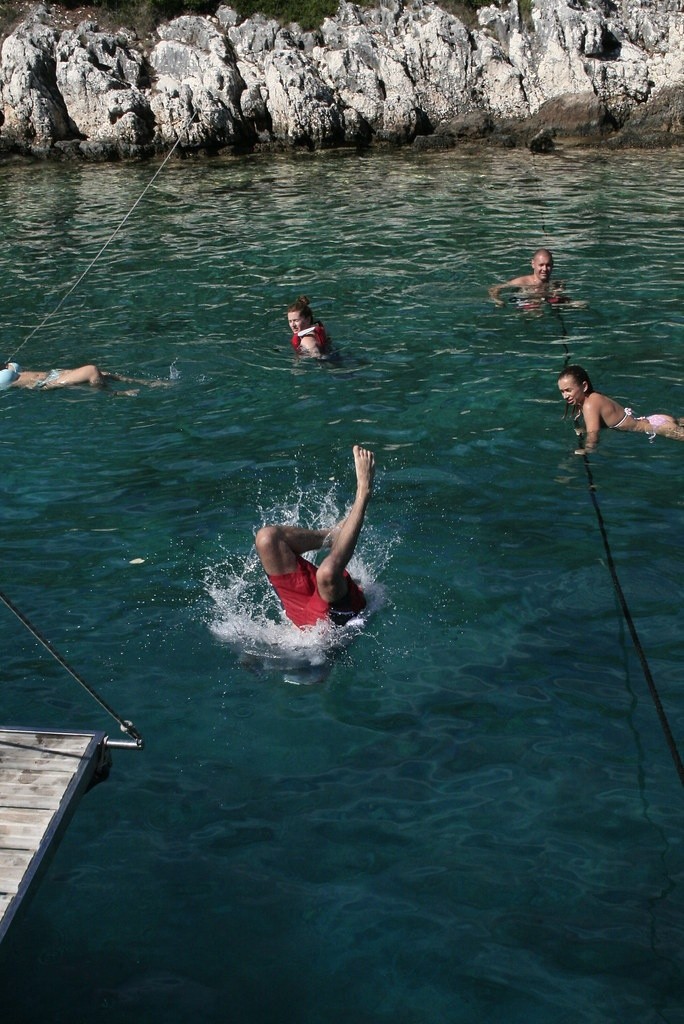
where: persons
[488,248,590,318]
[557,365,684,456]
[254,445,377,629]
[0,362,182,398]
[288,296,333,359]
